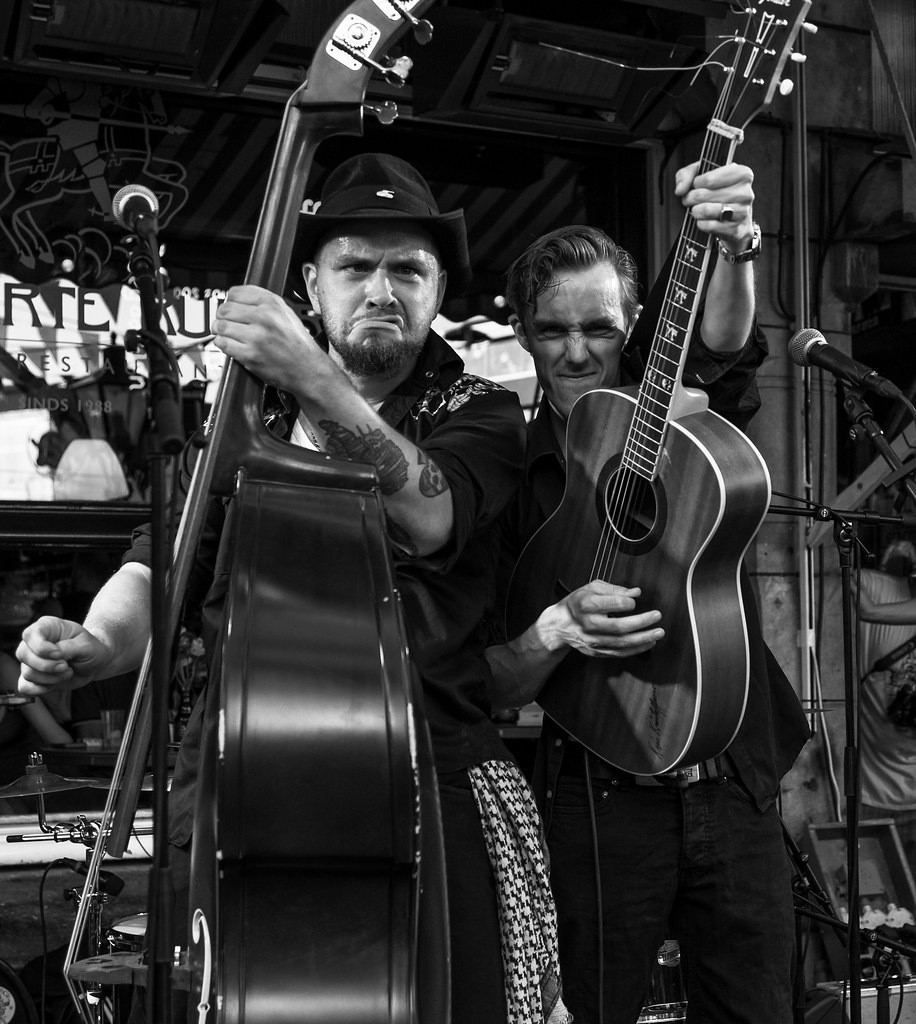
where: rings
[720,203,734,222]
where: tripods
[37,794,115,1024]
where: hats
[232,153,472,306]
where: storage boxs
[802,817,916,979]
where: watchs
[716,222,762,264]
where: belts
[570,754,732,787]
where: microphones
[112,183,159,237]
[787,327,902,400]
[62,858,125,897]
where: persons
[0,651,72,744]
[855,569,916,880]
[15,153,529,1024]
[377,160,851,1024]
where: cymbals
[90,768,175,795]
[0,766,96,799]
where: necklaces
[310,430,320,451]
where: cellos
[59,0,454,1024]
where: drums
[104,911,149,1024]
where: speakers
[816,975,916,1024]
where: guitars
[490,0,820,782]
